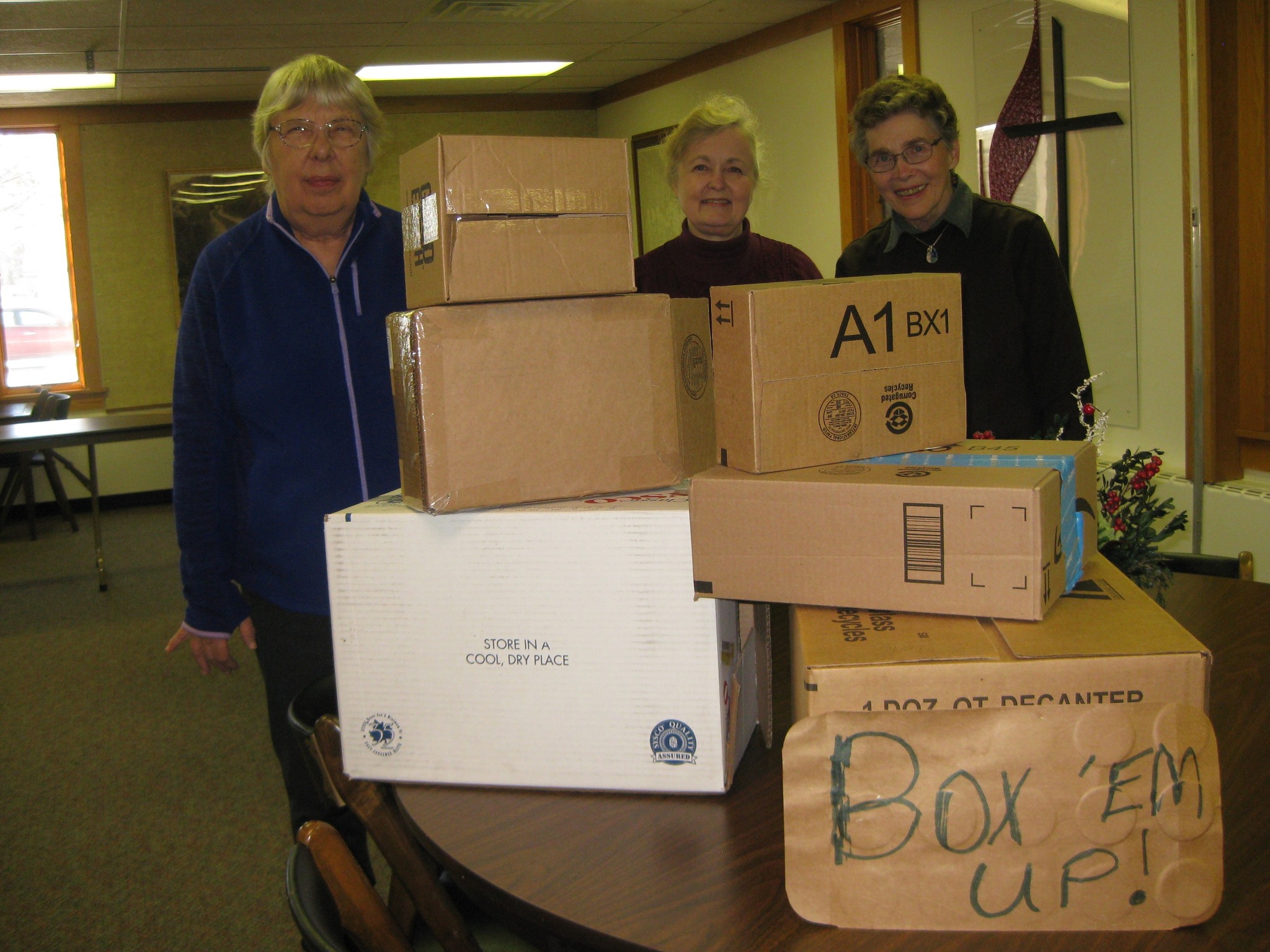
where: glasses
[864,136,942,173]
[269,118,369,149]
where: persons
[634,91,826,298]
[833,75,1093,441]
[165,53,407,952]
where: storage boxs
[323,130,1209,795]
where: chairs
[290,700,566,946]
[2,390,77,539]
[283,818,428,951]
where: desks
[370,572,1270,952]
[2,412,180,591]
